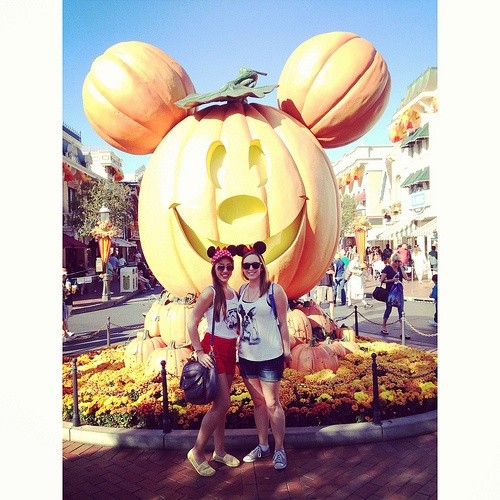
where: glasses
[393,260,401,263]
[242,262,262,270]
[216,265,233,271]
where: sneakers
[242,445,270,462]
[272,448,287,470]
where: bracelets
[196,349,204,354]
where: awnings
[400,168,430,187]
[400,124,429,149]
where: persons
[314,243,438,340]
[61,251,159,338]
[187,245,241,477]
[235,241,291,470]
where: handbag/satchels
[179,353,219,405]
[387,280,403,307]
[372,279,388,302]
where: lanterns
[137,72,341,304]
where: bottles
[394,272,400,279]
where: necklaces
[247,286,260,301]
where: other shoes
[399,333,410,339]
[364,303,373,309]
[213,451,241,467]
[66,329,75,336]
[187,448,216,476]
[381,328,389,334]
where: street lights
[96,204,111,302]
[354,202,367,272]
